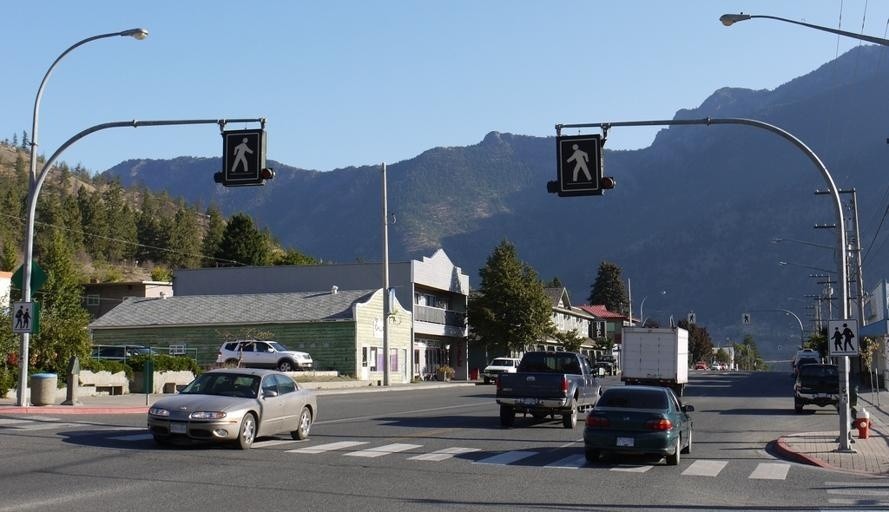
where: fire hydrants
[854,405,873,439]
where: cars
[695,360,729,371]
[146,367,318,450]
[584,393,695,465]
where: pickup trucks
[494,350,604,429]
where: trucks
[620,325,690,397]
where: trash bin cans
[31,373,58,406]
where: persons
[247,379,264,399]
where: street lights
[774,237,866,338]
[16,27,149,408]
[640,289,667,328]
[703,6,889,80]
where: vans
[792,348,821,377]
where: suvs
[483,357,521,385]
[792,364,840,414]
[216,340,313,372]
[594,354,619,377]
